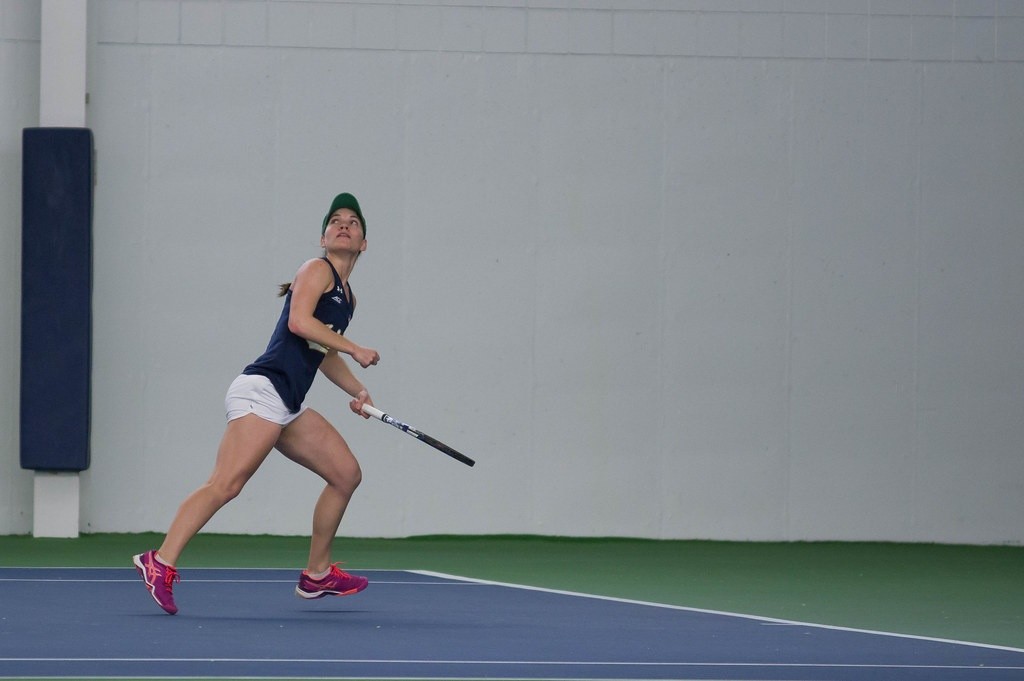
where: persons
[132,191,379,615]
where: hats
[322,193,367,239]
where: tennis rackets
[361,403,476,468]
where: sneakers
[132,550,181,615]
[295,561,369,600]
